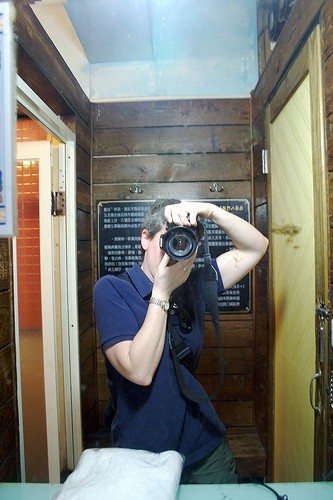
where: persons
[91,198,269,484]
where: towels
[55,447,186,500]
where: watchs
[148,298,170,311]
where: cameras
[159,219,198,267]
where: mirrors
[1,1,331,487]
